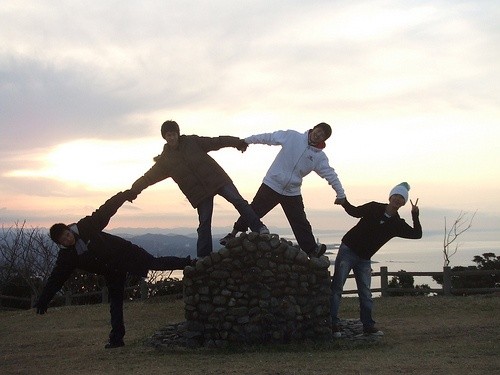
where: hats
[389,182,410,206]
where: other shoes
[259,226,269,234]
[219,233,235,245]
[309,243,327,259]
[327,325,342,338]
[104,341,125,348]
[363,327,384,335]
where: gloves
[33,297,49,315]
[236,139,249,151]
[123,189,137,203]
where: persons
[34,188,199,349]
[220,124,346,259]
[335,182,423,339]
[130,120,271,263]
